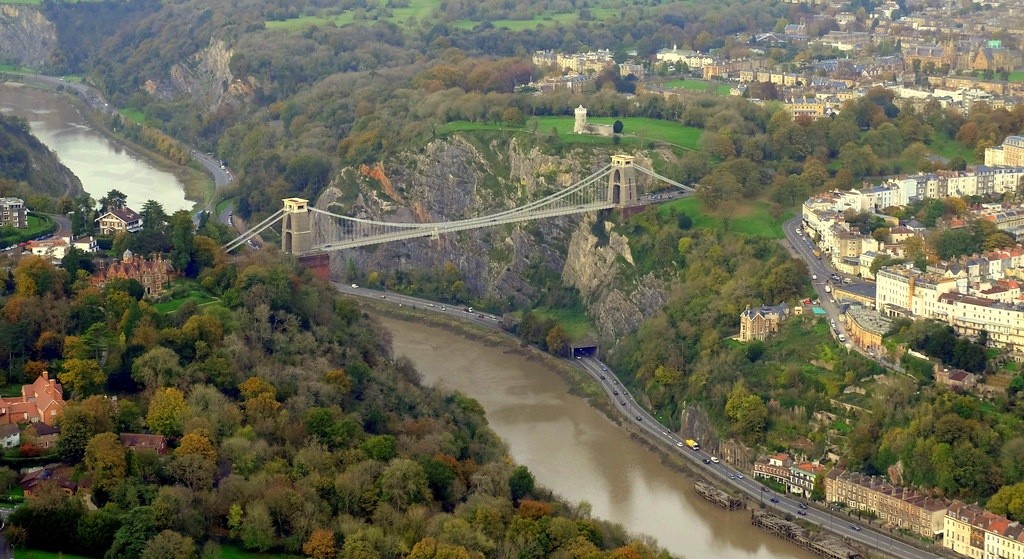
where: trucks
[685,439,699,451]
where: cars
[601,376,605,379]
[614,391,618,395]
[771,498,778,502]
[762,486,768,492]
[636,415,642,421]
[602,368,606,370]
[825,285,830,292]
[824,319,846,341]
[703,459,709,464]
[850,524,860,531]
[621,401,625,405]
[427,302,497,321]
[728,474,735,479]
[613,379,617,384]
[711,456,719,464]
[813,274,817,280]
[736,474,744,478]
[677,442,684,447]
[796,228,820,258]
[575,348,589,359]
[800,503,808,509]
[797,510,806,515]
